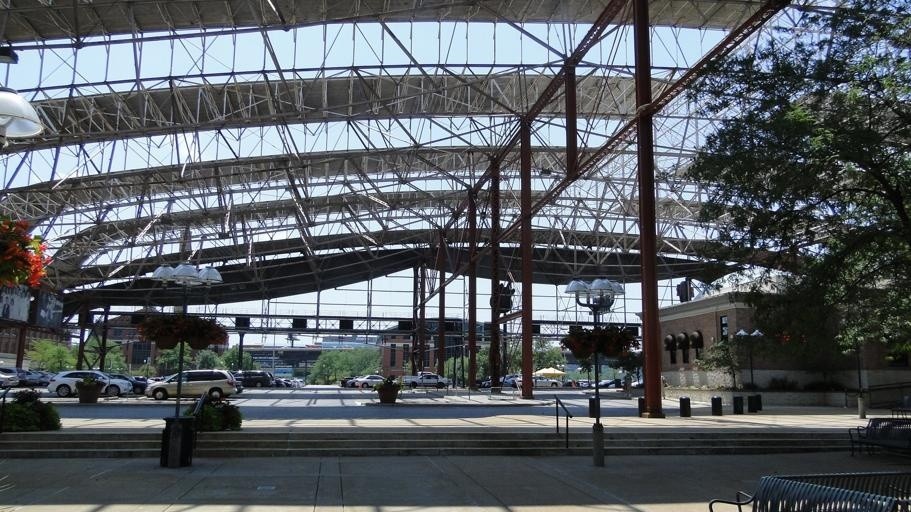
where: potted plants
[73,372,103,405]
[369,371,407,405]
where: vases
[186,336,211,350]
[153,332,179,350]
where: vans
[144,370,236,399]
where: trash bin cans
[160,416,197,468]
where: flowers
[0,210,57,298]
[133,309,187,342]
[180,312,232,344]
[594,320,641,357]
[558,323,600,358]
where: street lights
[561,365,564,372]
[231,362,233,369]
[149,260,223,470]
[322,363,326,385]
[143,359,146,365]
[562,276,624,466]
[736,328,765,385]
[556,365,559,370]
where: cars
[232,370,304,390]
[0,366,167,398]
[482,374,642,390]
[340,371,453,390]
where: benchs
[704,408,910,512]
